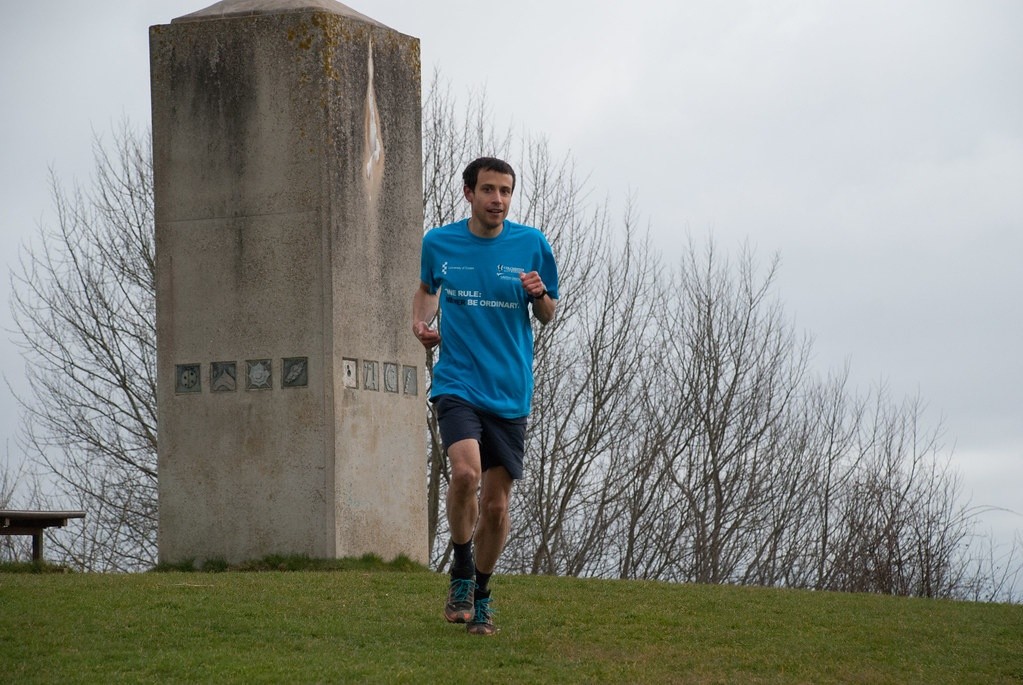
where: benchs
[0,509,85,561]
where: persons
[412,157,560,635]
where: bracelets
[535,284,547,298]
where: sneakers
[444,575,479,623]
[467,589,495,636]
